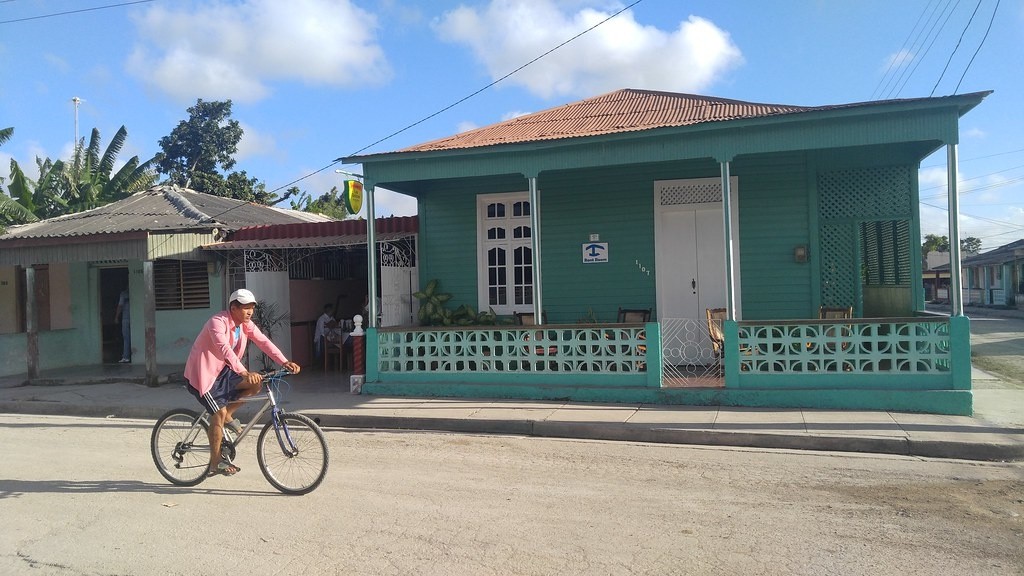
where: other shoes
[119,358,130,362]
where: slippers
[224,417,244,436]
[210,461,237,474]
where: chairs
[511,308,559,371]
[606,306,652,373]
[804,304,855,373]
[706,307,761,374]
[322,319,346,374]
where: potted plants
[467,307,500,370]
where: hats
[229,288,258,306]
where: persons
[115,286,131,364]
[314,289,382,372]
[183,290,300,476]
